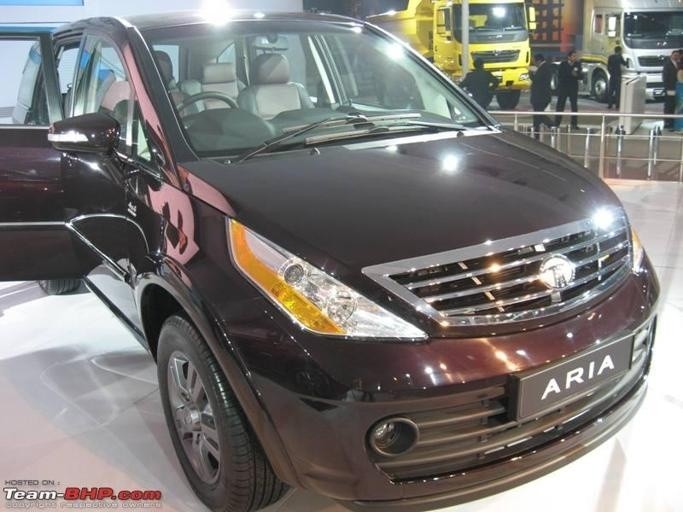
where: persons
[456,58,499,112]
[524,53,555,141]
[553,50,586,130]
[663,47,683,136]
[606,46,629,110]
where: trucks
[363,0,533,110]
[525,0,683,105]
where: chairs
[235,53,317,122]
[154,50,199,130]
[200,62,240,111]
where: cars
[0,10,665,512]
